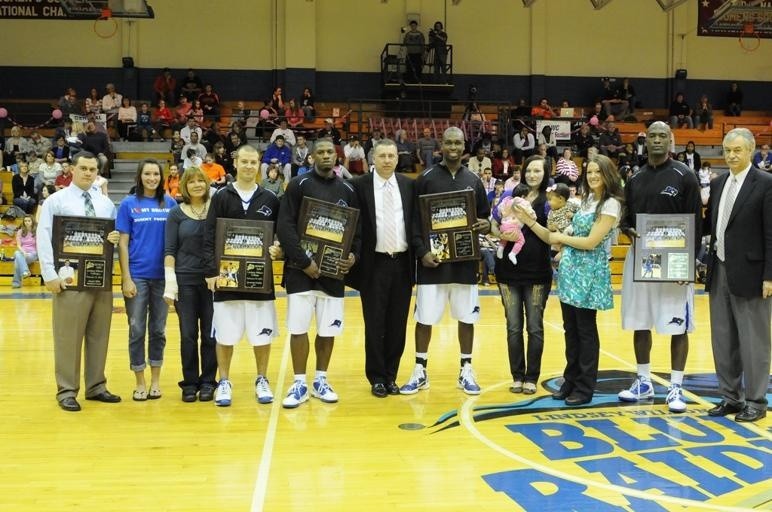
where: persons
[162,166,220,402]
[37,151,120,411]
[429,21,448,84]
[548,153,625,405]
[2,66,317,287]
[346,139,416,399]
[618,121,703,413]
[276,137,362,409]
[701,128,772,423]
[115,157,178,401]
[459,76,772,288]
[398,126,492,395]
[319,117,444,173]
[200,145,283,407]
[403,20,425,84]
[490,155,552,395]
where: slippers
[147,386,161,400]
[133,389,149,401]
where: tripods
[417,41,448,82]
[462,100,484,121]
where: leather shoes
[708,400,743,416]
[371,383,387,397]
[565,395,586,405]
[735,406,766,421]
[384,380,399,394]
[552,390,568,399]
[58,397,80,411]
[85,390,121,402]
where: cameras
[429,28,438,40]
[468,82,480,99]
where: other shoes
[523,383,536,394]
[509,381,523,392]
[182,389,197,402]
[199,387,214,400]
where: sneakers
[399,364,429,394]
[214,379,232,406]
[665,383,687,412]
[21,272,30,279]
[282,380,309,407]
[255,375,274,403]
[618,375,654,401]
[311,376,338,401]
[12,283,19,288]
[457,362,481,394]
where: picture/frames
[418,189,481,263]
[287,195,360,280]
[632,211,696,283]
[214,217,274,294]
[52,214,115,290]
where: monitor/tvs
[561,107,575,118]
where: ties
[716,176,737,262]
[382,182,396,255]
[81,192,96,218]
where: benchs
[608,230,632,288]
[0,170,43,286]
